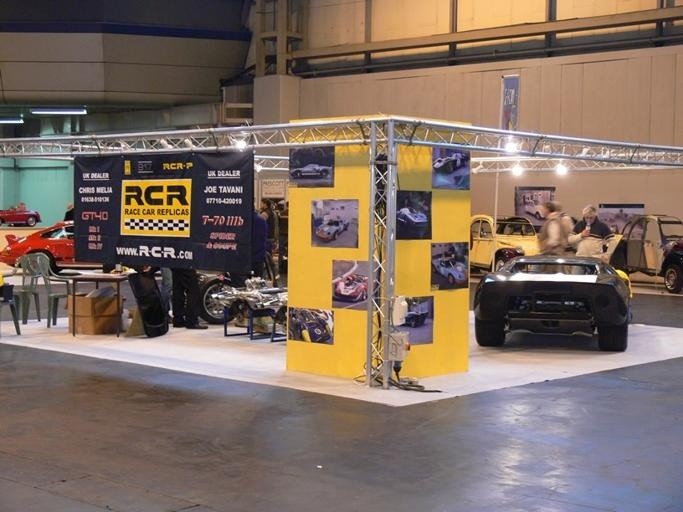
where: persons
[270,201,279,215]
[170,267,208,329]
[159,268,172,323]
[259,199,280,287]
[536,202,565,256]
[567,204,614,264]
[229,206,269,334]
[551,200,574,257]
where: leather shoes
[173,323,208,328]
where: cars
[432,256,467,286]
[196,272,288,325]
[524,199,561,219]
[316,219,350,241]
[611,213,682,294]
[405,311,430,328]
[471,214,538,274]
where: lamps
[0,116,24,124]
[29,108,87,115]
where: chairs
[0,284,22,337]
[34,251,71,327]
[10,253,41,325]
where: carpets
[0,310,682,408]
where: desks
[64,271,126,337]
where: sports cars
[0,206,42,227]
[0,221,106,276]
[290,310,331,342]
[291,162,332,180]
[335,272,369,303]
[472,254,634,351]
[433,150,468,174]
[397,206,429,227]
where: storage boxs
[65,292,124,316]
[69,314,119,336]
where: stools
[222,298,287,342]
